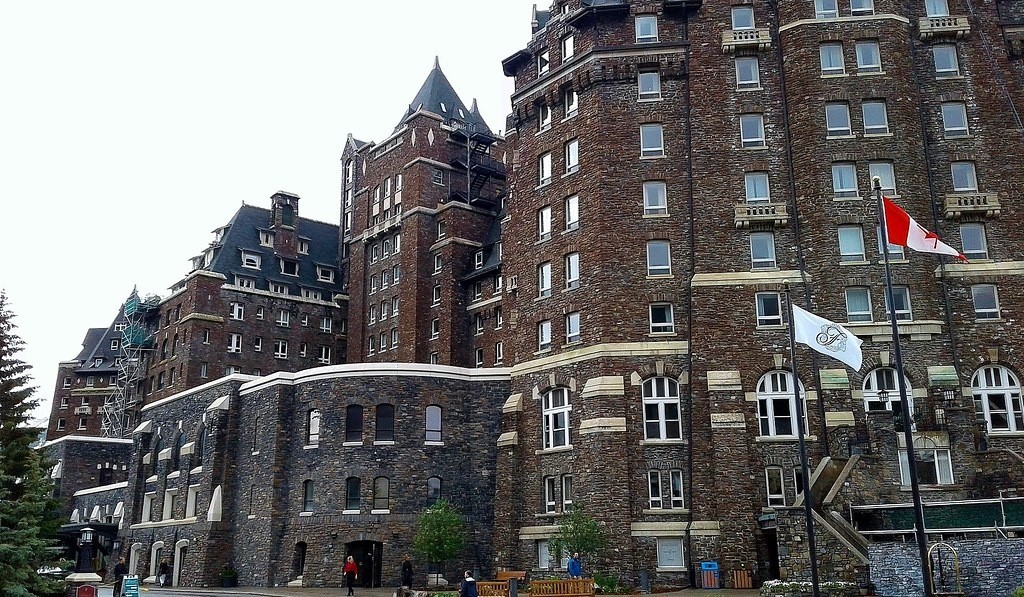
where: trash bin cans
[702,562,720,589]
[734,571,753,589]
[66,572,103,597]
[692,562,702,589]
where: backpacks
[567,562,570,573]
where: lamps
[941,388,956,408]
[877,389,889,404]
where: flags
[882,193,970,263]
[792,301,865,373]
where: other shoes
[161,584,163,588]
[347,589,354,596]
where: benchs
[493,571,528,589]
[528,579,597,597]
[475,580,510,597]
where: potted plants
[859,584,868,595]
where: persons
[400,553,415,589]
[458,570,478,597]
[155,557,168,587]
[343,556,358,596]
[568,551,582,580]
[113,556,126,597]
